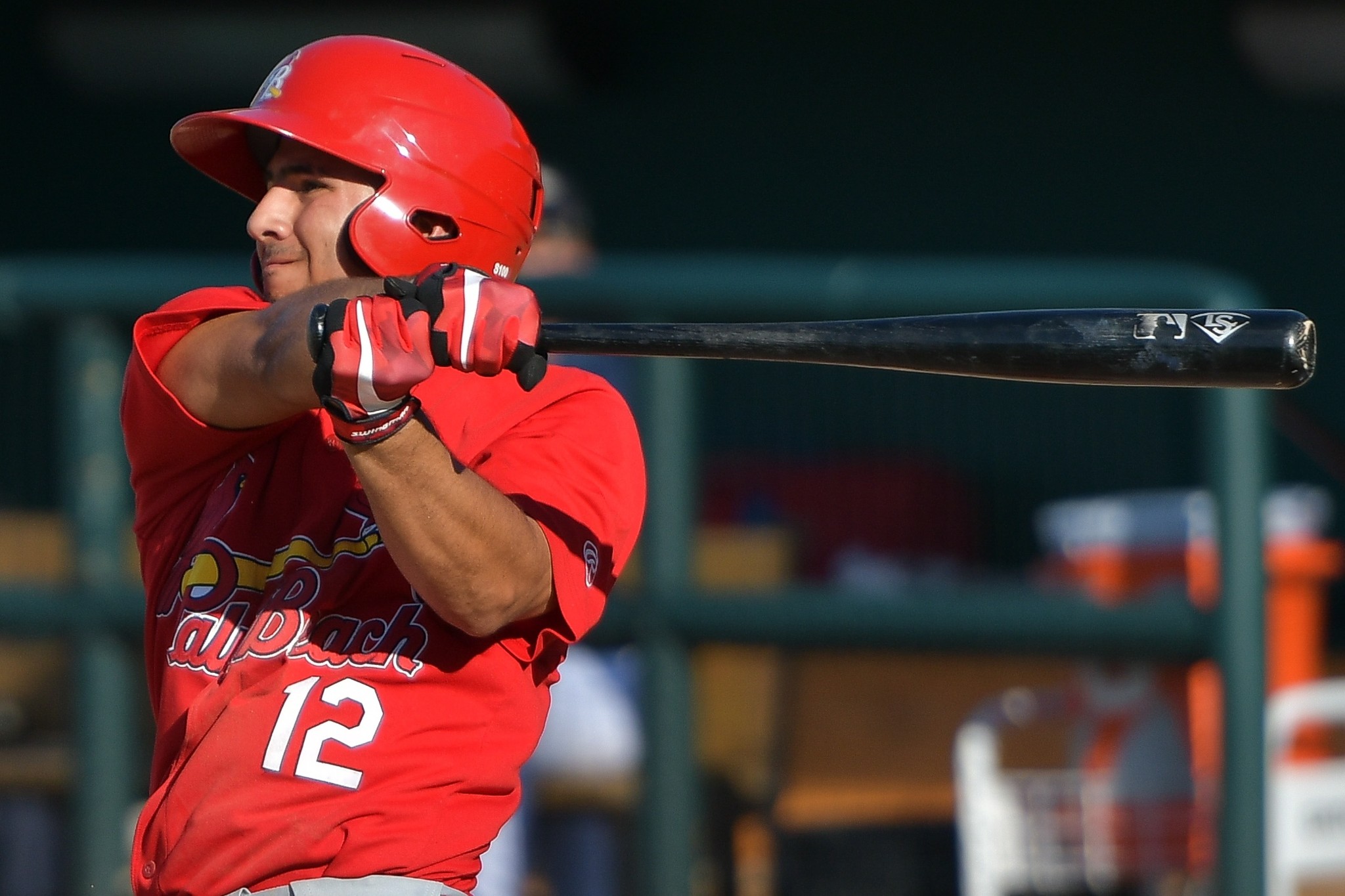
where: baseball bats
[307,303,1318,391]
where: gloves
[307,292,433,446]
[382,253,547,394]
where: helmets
[171,34,549,292]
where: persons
[125,35,645,896]
